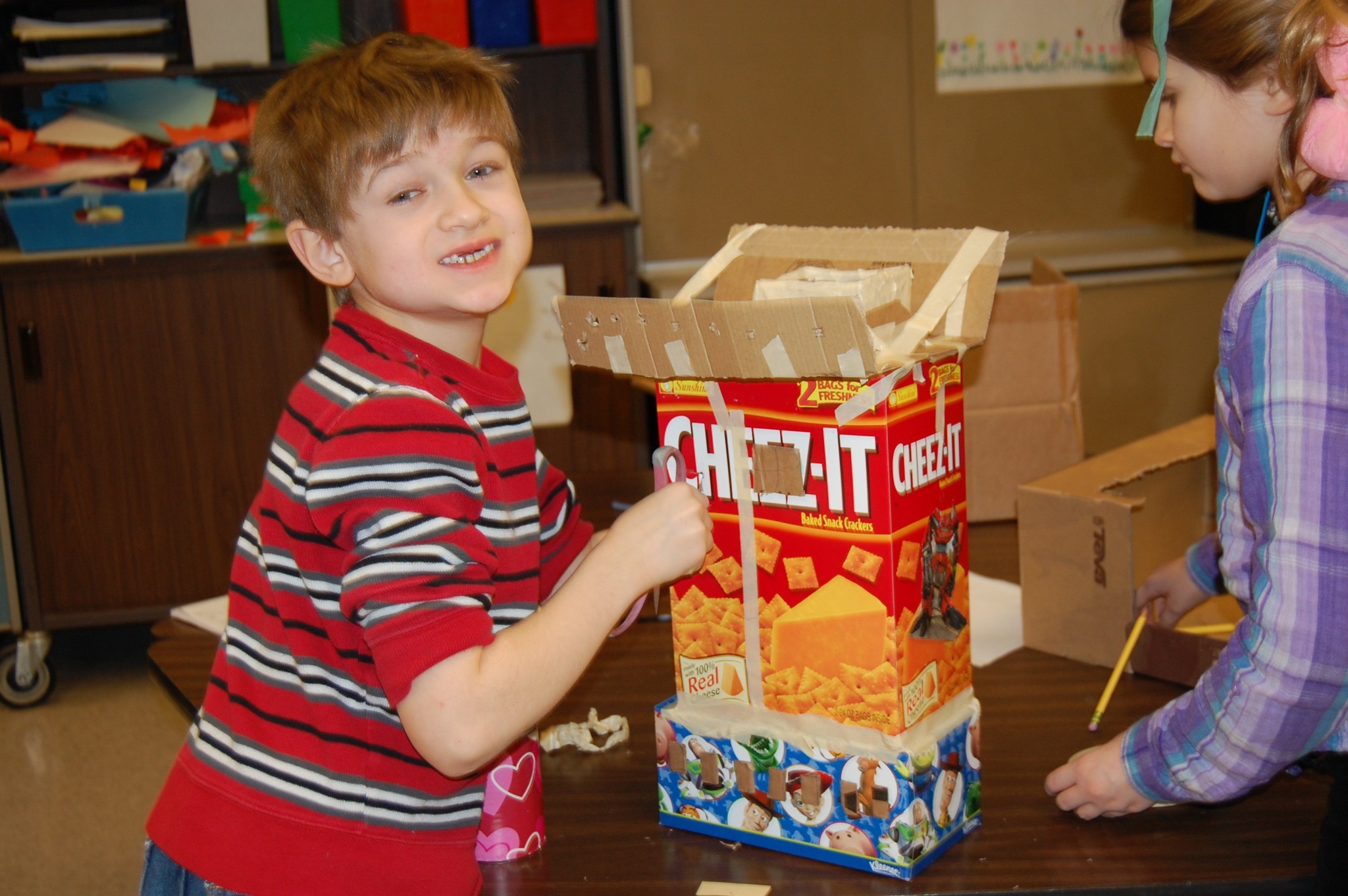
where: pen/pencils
[1086,605,1148,731]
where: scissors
[604,445,686,638]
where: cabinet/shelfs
[0,200,656,642]
[0,1,643,261]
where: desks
[144,600,1348,896]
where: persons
[1037,1,1348,896]
[137,32,715,896]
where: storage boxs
[650,695,982,883]
[652,353,972,734]
[1015,416,1220,676]
[963,247,1089,527]
[1,177,194,254]
